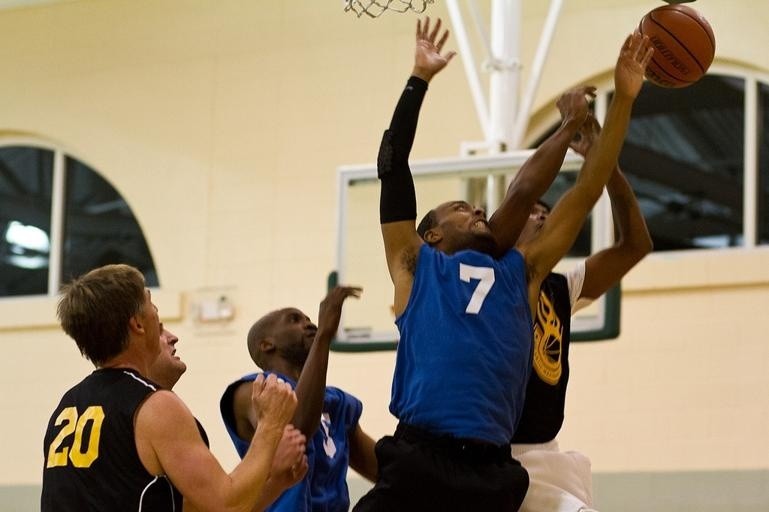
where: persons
[40,262,297,512]
[145,322,311,512]
[350,14,654,510]
[490,84,653,512]
[218,284,378,511]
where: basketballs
[635,5,715,88]
